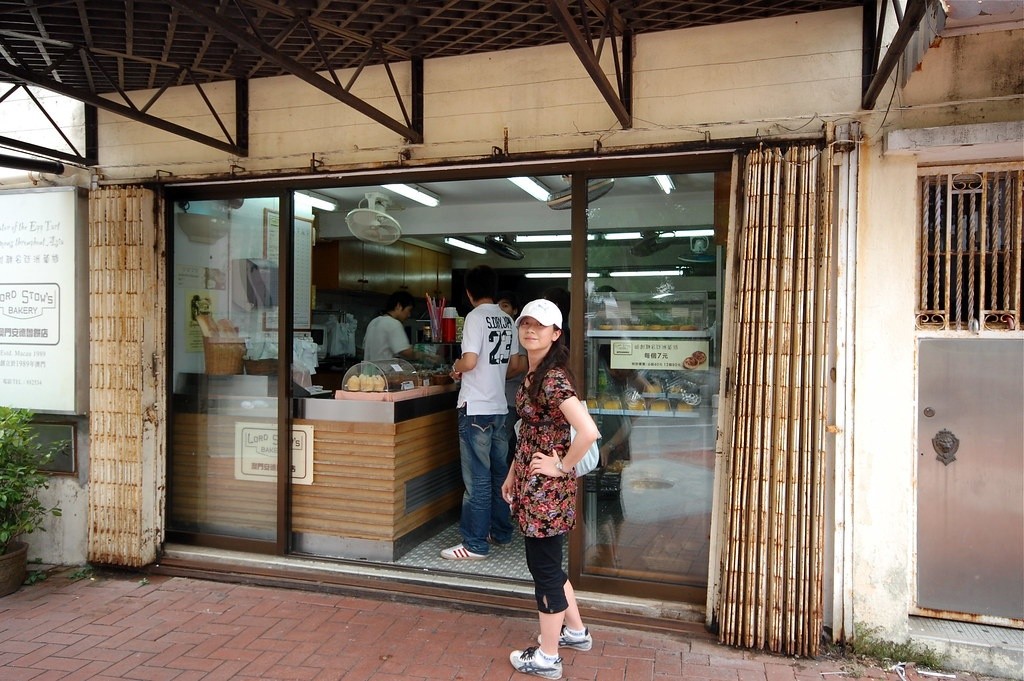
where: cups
[430,319,442,343]
[443,318,457,343]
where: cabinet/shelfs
[312,237,452,302]
[586,328,715,419]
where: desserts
[347,375,385,391]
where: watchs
[557,460,568,475]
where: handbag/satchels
[514,399,602,477]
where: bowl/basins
[432,372,451,384]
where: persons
[363,292,444,374]
[583,344,655,570]
[543,289,570,349]
[502,298,598,678]
[498,298,517,319]
[441,267,513,558]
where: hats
[514,298,564,330]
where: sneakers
[537,625,593,651]
[487,532,512,548]
[509,645,564,680]
[440,543,486,560]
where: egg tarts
[684,357,700,369]
[600,325,697,332]
[693,351,706,363]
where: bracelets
[607,440,615,452]
[452,364,460,374]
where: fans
[546,172,615,209]
[678,235,716,264]
[485,232,526,262]
[629,229,677,258]
[345,191,403,245]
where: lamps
[509,175,554,202]
[381,182,441,208]
[444,234,488,255]
[294,188,339,212]
[654,174,677,194]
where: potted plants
[0,404,72,598]
